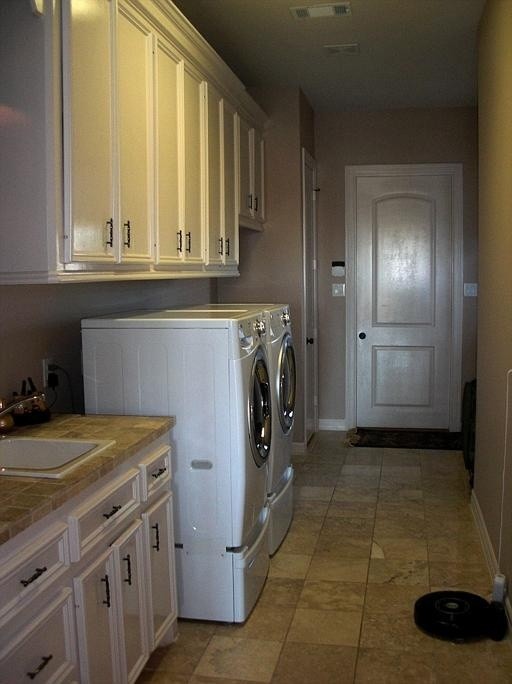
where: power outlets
[42,358,62,389]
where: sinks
[0,438,99,472]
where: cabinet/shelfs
[238,76,266,233]
[51,447,151,683]
[0,1,154,286]
[0,499,80,684]
[206,40,240,278]
[125,416,179,654]
[154,1,206,280]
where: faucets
[1,391,47,439]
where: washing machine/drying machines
[164,302,298,557]
[78,308,271,628]
